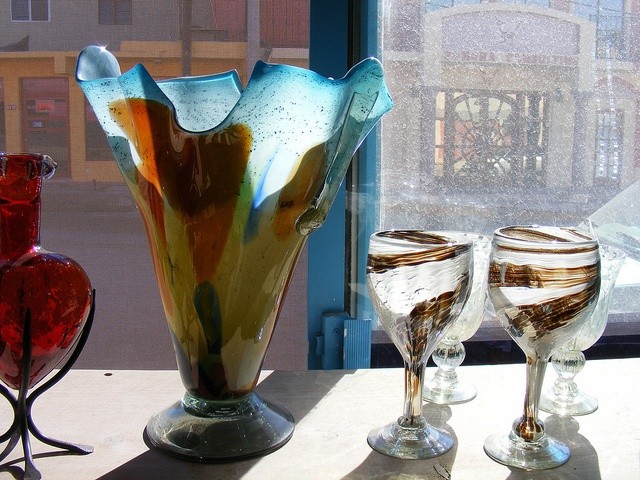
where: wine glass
[364,231,474,462]
[483,223,602,469]
[421,231,491,403]
[532,243,626,419]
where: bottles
[0,147,94,391]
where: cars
[575,174,640,318]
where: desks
[0,357,640,480]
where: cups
[69,38,395,466]
